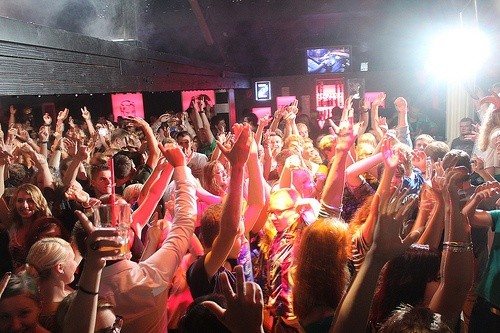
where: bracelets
[362,109,368,112]
[200,110,205,113]
[40,141,47,144]
[442,241,473,252]
[78,286,98,295]
[317,201,343,219]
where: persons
[0,83,500,333]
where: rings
[90,242,99,249]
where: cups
[91,203,131,260]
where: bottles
[318,94,342,120]
[424,157,434,186]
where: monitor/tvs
[305,45,354,74]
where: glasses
[266,206,294,216]
[217,170,226,174]
[98,315,124,333]
